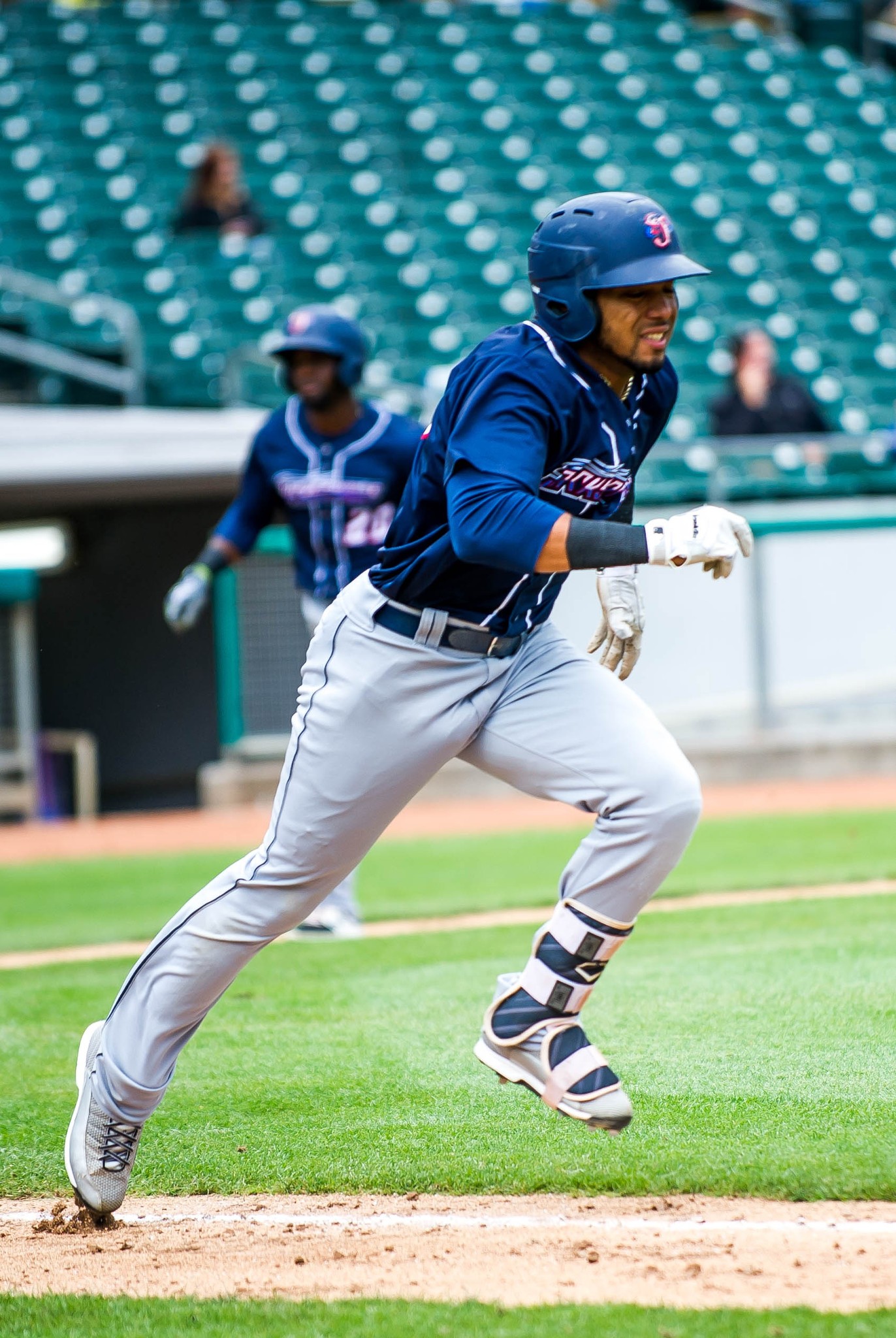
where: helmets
[526,192,713,342]
[265,302,364,388]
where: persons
[169,148,263,236]
[58,190,754,1212]
[163,306,424,936]
[715,320,828,440]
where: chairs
[0,1,896,498]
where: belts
[374,602,521,658]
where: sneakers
[471,974,633,1129]
[62,1020,144,1214]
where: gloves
[164,570,208,633]
[643,504,754,578]
[588,564,646,680]
